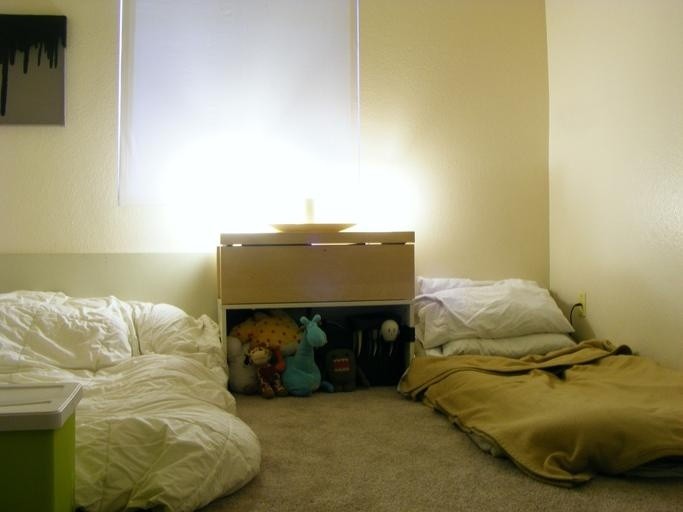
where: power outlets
[577,291,586,318]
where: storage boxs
[0,382,83,511]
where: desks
[215,232,416,375]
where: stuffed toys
[224,311,406,401]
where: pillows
[443,331,578,357]
